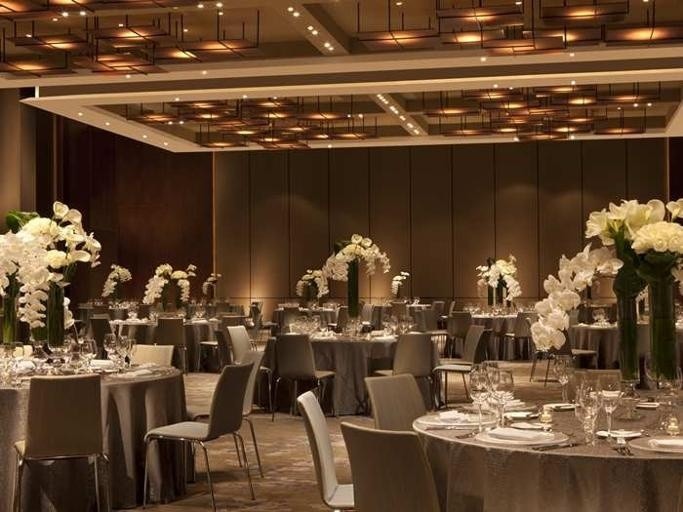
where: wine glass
[481,361,499,432]
[468,366,492,435]
[598,379,620,447]
[487,367,515,431]
[573,391,599,445]
[591,307,607,326]
[554,354,576,404]
[0,338,98,385]
[650,367,683,435]
[582,377,604,447]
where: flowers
[0,197,102,343]
[295,233,410,298]
[100,263,221,305]
[473,197,683,352]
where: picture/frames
[649,268,674,380]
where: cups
[103,332,138,376]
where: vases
[615,293,641,382]
[347,260,358,319]
[45,284,65,354]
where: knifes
[426,425,480,431]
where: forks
[455,426,497,440]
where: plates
[416,413,497,426]
[510,422,554,429]
[589,390,624,399]
[475,428,571,446]
[596,430,642,438]
[543,403,576,410]
[504,411,540,420]
[628,436,683,453]
